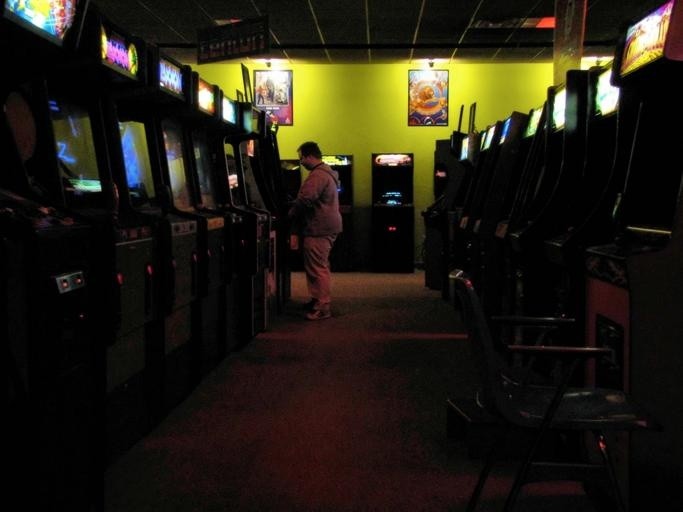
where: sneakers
[302,297,332,321]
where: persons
[287,140,345,322]
[255,83,267,107]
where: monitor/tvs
[46,100,104,195]
[191,130,216,196]
[224,143,240,190]
[276,170,301,202]
[117,119,157,203]
[330,166,352,204]
[162,125,193,194]
[374,167,413,204]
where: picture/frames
[406,68,450,128]
[250,69,294,128]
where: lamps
[263,58,273,68]
[425,56,435,69]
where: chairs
[444,262,654,511]
